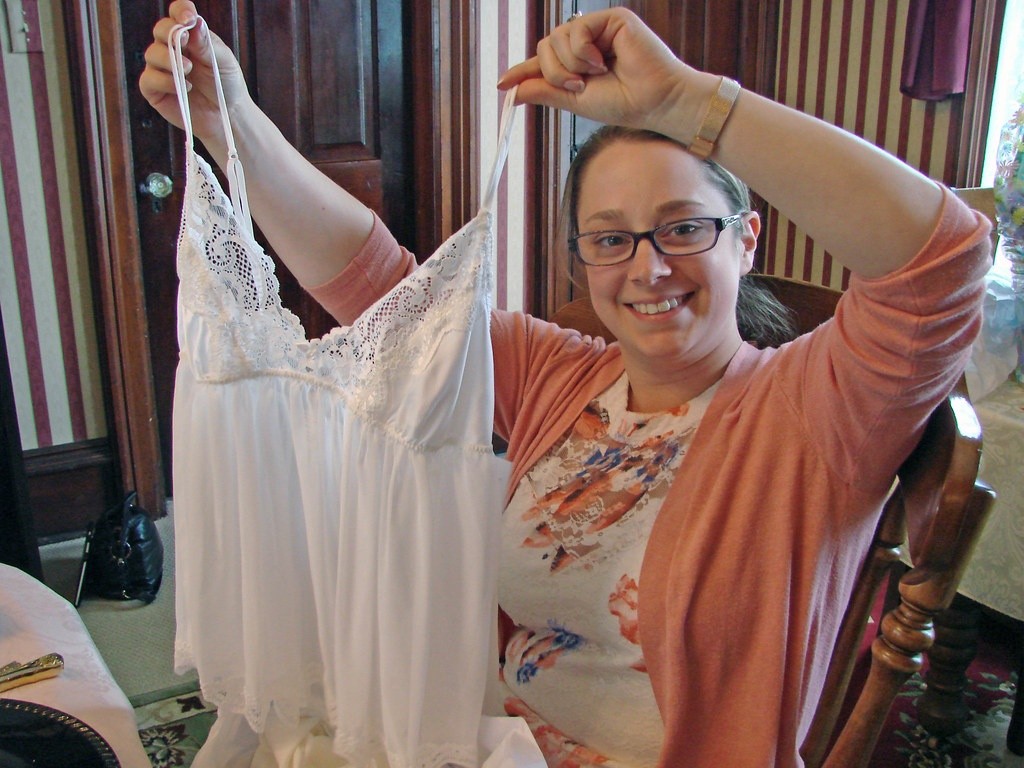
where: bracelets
[689,76,740,161]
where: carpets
[121,565,1024,768]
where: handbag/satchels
[76,491,164,603]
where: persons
[139,0,993,767]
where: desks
[893,377,1024,760]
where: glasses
[568,211,743,266]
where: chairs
[489,276,1000,768]
